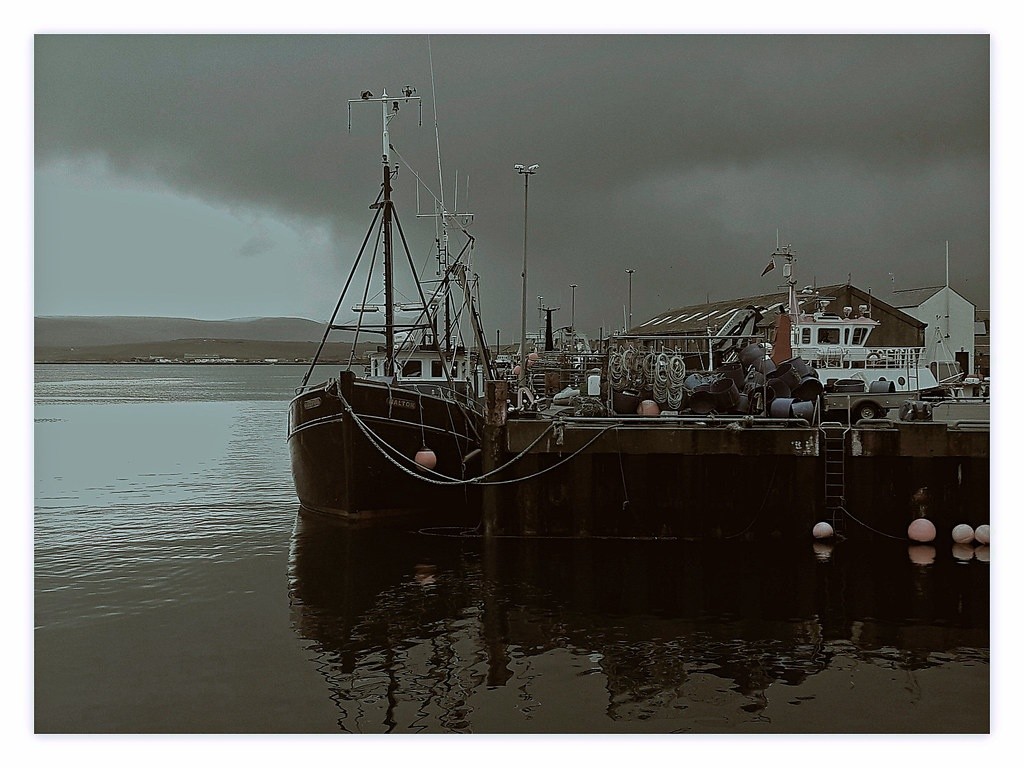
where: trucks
[826,392,920,426]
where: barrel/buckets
[612,342,895,426]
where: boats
[286,87,498,538]
[517,294,597,401]
[281,505,473,733]
[710,226,944,398]
